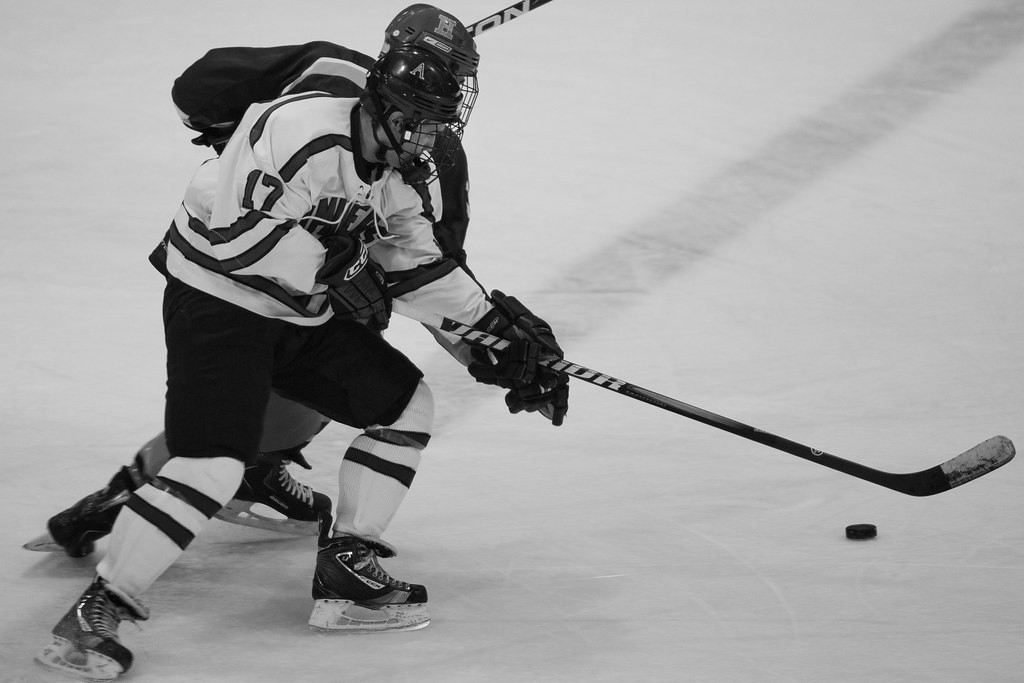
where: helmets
[362,47,464,125]
[378,3,479,80]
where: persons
[34,44,559,683]
[26,3,571,556]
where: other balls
[846,522,878,542]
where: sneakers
[212,455,333,536]
[310,512,434,633]
[35,580,151,681]
[22,466,138,558]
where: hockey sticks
[386,294,1017,500]
[464,0,553,38]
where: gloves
[466,290,570,427]
[314,232,392,333]
